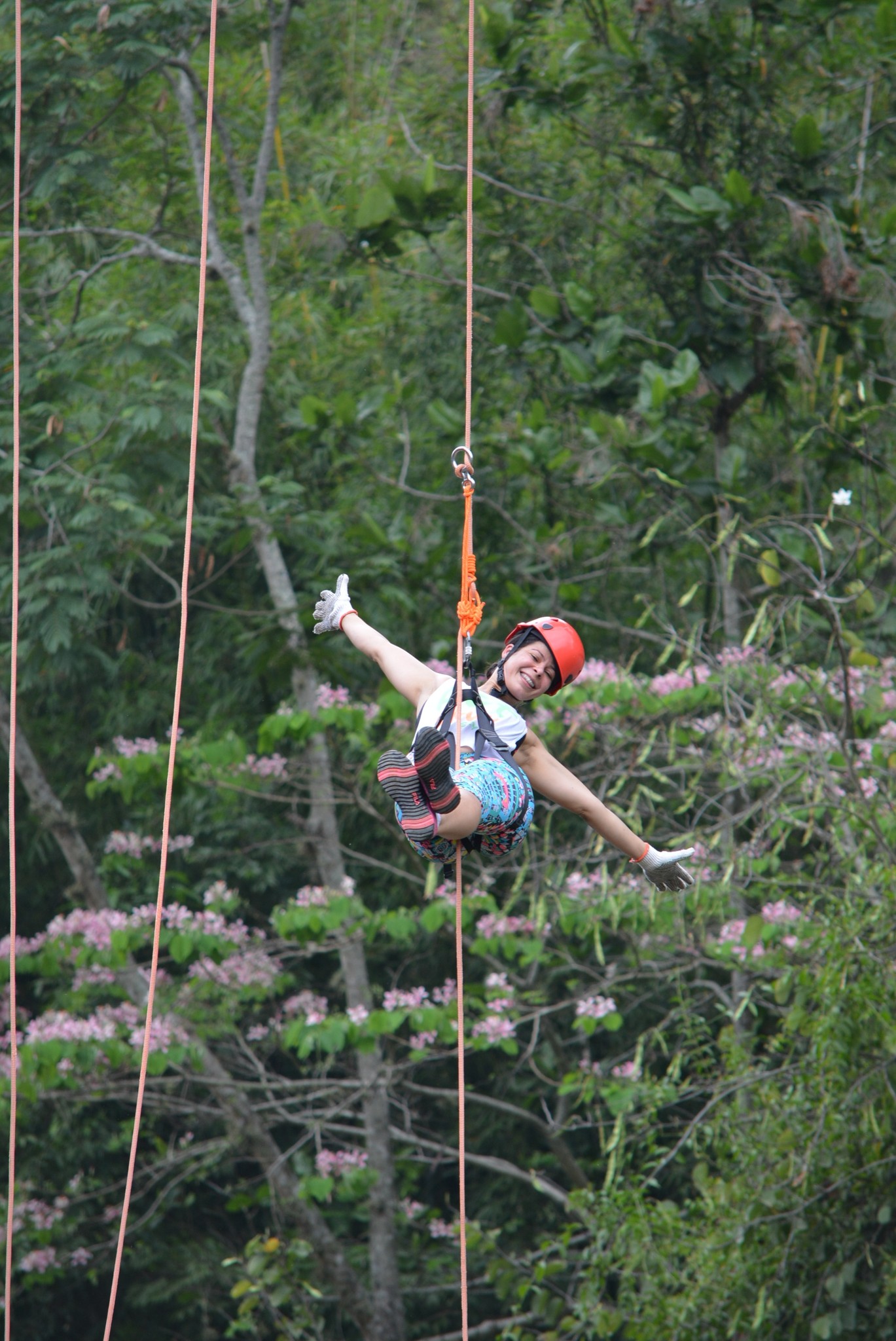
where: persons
[312,575,696,891]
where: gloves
[628,840,695,893]
[312,575,357,634]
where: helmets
[505,616,586,696]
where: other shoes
[376,749,434,842]
[412,726,461,815]
